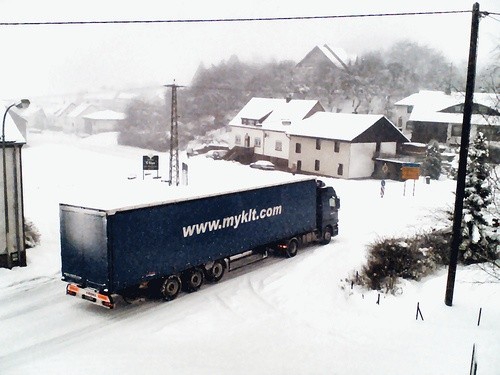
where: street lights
[0,98,31,269]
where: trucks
[57,173,343,312]
